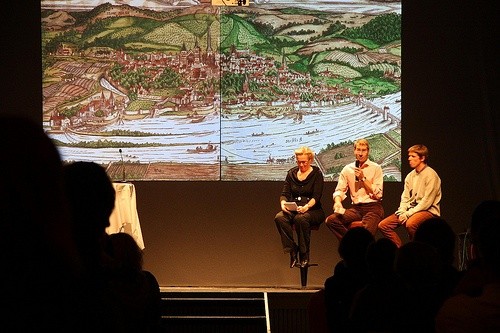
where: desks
[106,183,145,250]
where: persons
[366,238,402,285]
[434,200,500,333]
[0,112,159,333]
[394,241,439,333]
[327,227,375,333]
[325,138,383,237]
[348,285,435,333]
[414,219,466,290]
[378,144,442,247]
[275,147,325,268]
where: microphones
[355,159,361,183]
[119,149,127,182]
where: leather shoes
[299,251,308,267]
[289,244,298,268]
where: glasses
[296,159,309,164]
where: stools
[347,221,364,229]
[289,224,321,290]
